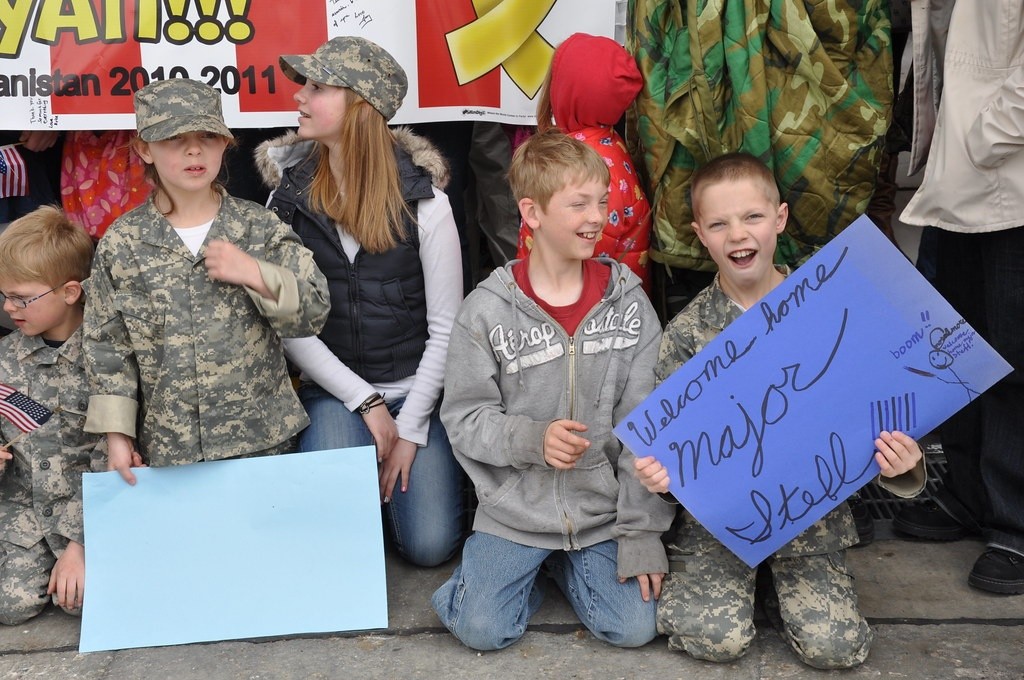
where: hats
[278,35,408,121]
[133,78,234,143]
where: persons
[520,31,651,289]
[255,36,471,570]
[82,78,330,485]
[431,129,677,646]
[0,129,157,241]
[633,153,926,670]
[0,206,108,625]
[895,0,1024,594]
[849,123,900,547]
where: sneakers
[892,498,971,542]
[846,491,875,545]
[967,547,1024,595]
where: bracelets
[358,393,386,414]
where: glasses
[0,280,70,309]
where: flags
[0,383,52,433]
[0,145,28,199]
